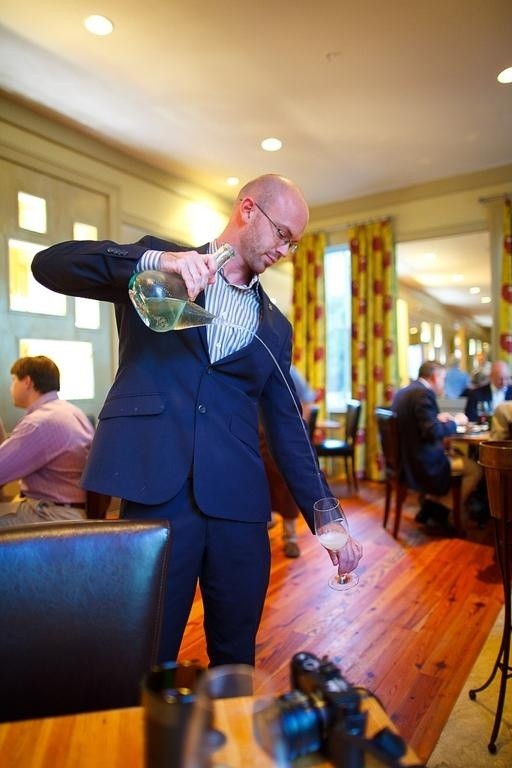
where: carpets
[424,601,511,768]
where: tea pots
[127,242,237,333]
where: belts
[54,503,84,508]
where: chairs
[1,515,173,719]
[375,407,470,539]
[307,406,318,440]
[317,398,362,492]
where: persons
[29,173,364,696]
[256,364,318,563]
[471,350,493,385]
[465,358,512,521]
[0,356,98,531]
[444,358,471,398]
[459,369,488,396]
[389,361,483,537]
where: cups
[144,662,209,768]
[476,401,487,426]
[181,662,286,768]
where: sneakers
[413,502,456,537]
[282,535,300,559]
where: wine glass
[313,497,359,593]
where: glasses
[254,201,300,254]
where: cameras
[253,649,368,767]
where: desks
[1,684,430,767]
[445,428,496,443]
